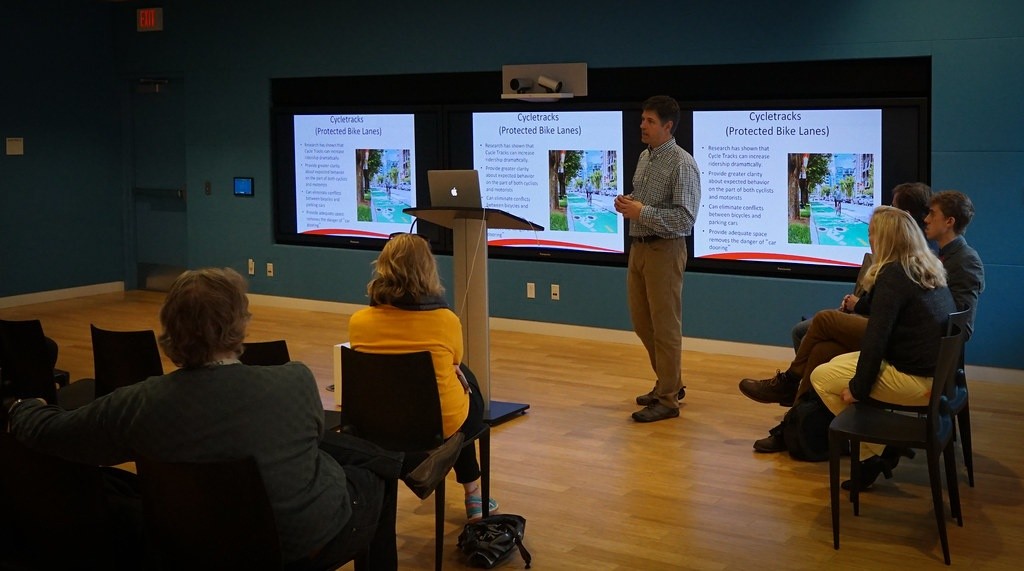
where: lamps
[538,75,563,93]
[510,78,533,94]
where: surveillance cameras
[510,78,533,92]
[538,75,562,93]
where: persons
[350,234,499,519]
[385,176,392,194]
[614,95,700,424]
[586,179,593,202]
[792,181,934,357]
[832,185,845,212]
[9,269,465,571]
[810,204,960,492]
[739,189,985,454]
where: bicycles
[833,196,841,216]
[386,184,392,200]
[585,189,592,206]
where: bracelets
[9,398,23,413]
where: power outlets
[267,262,273,276]
[551,283,560,301]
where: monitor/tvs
[274,97,927,278]
[234,176,254,195]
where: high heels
[842,455,893,489]
[880,444,916,471]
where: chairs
[829,304,975,565]
[0,319,489,571]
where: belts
[637,235,660,243]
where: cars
[809,191,874,206]
[567,185,617,197]
[370,182,411,191]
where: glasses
[388,232,430,244]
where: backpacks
[786,391,849,461]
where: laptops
[428,170,482,208]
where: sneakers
[636,386,685,404]
[465,495,498,519]
[739,369,796,408]
[754,425,787,452]
[633,402,679,422]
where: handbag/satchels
[457,514,531,569]
